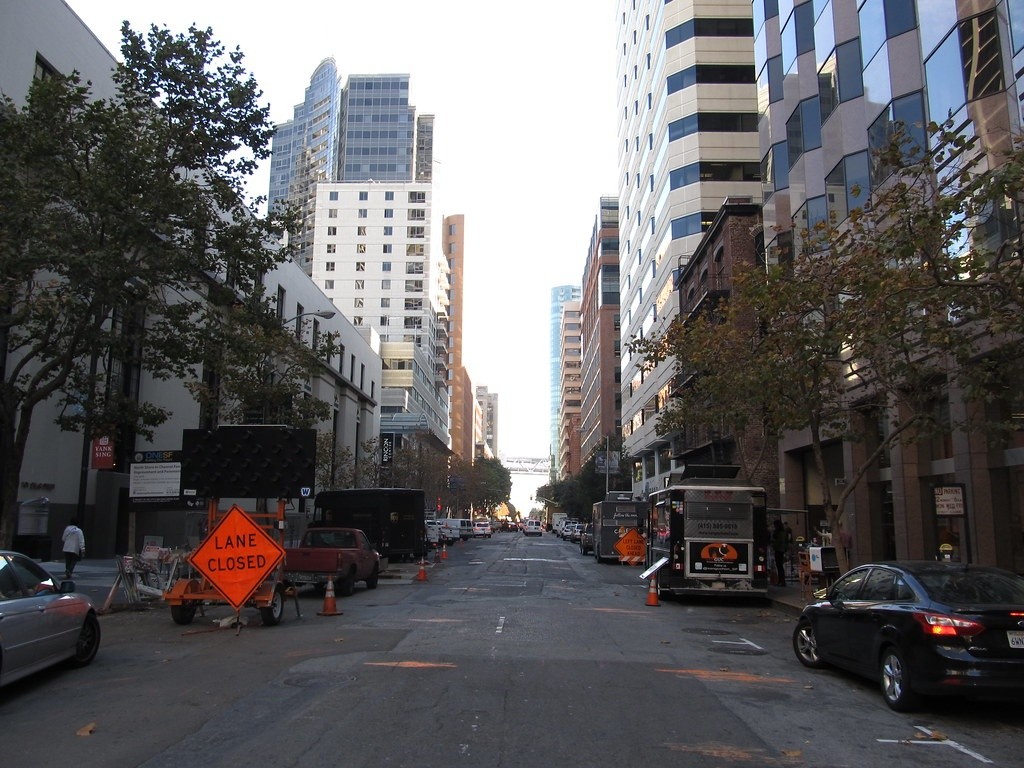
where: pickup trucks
[281,524,387,596]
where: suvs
[520,519,544,536]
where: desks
[797,569,841,601]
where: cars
[792,560,1024,713]
[552,519,596,554]
[0,551,101,691]
[428,515,518,546]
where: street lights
[576,428,609,495]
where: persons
[62,518,85,578]
[768,520,793,587]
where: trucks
[648,477,770,605]
[311,488,431,556]
[551,512,568,527]
[592,498,651,564]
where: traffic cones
[770,559,778,586]
[646,571,661,606]
[441,544,449,558]
[316,573,343,617]
[416,555,428,581]
[432,547,441,563]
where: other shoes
[66,570,71,577]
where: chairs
[312,534,330,548]
